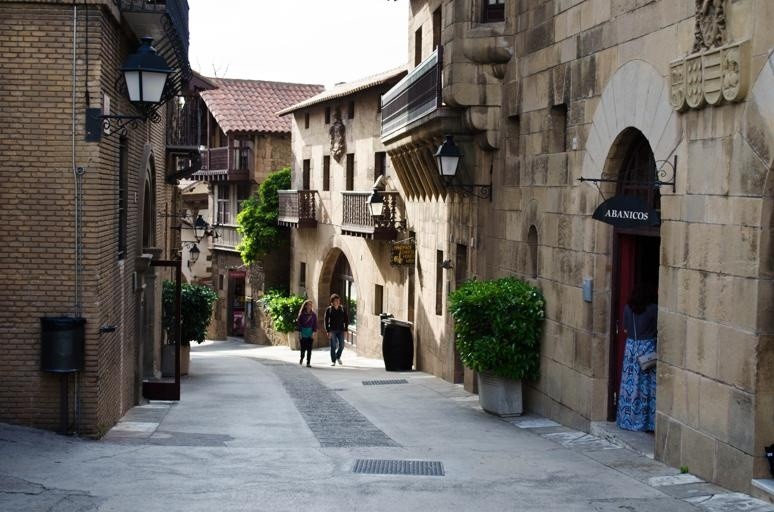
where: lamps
[187,213,208,267]
[365,190,406,231]
[433,136,492,204]
[82,37,176,143]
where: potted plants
[268,292,307,351]
[160,279,219,377]
[444,273,545,418]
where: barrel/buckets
[382,322,414,371]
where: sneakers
[307,363,312,367]
[338,359,342,364]
[331,362,335,366]
[300,359,302,364]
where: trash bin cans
[380,317,414,373]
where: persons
[614,284,658,433]
[324,294,349,365]
[297,299,317,367]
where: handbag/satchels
[301,327,313,338]
[638,352,656,371]
